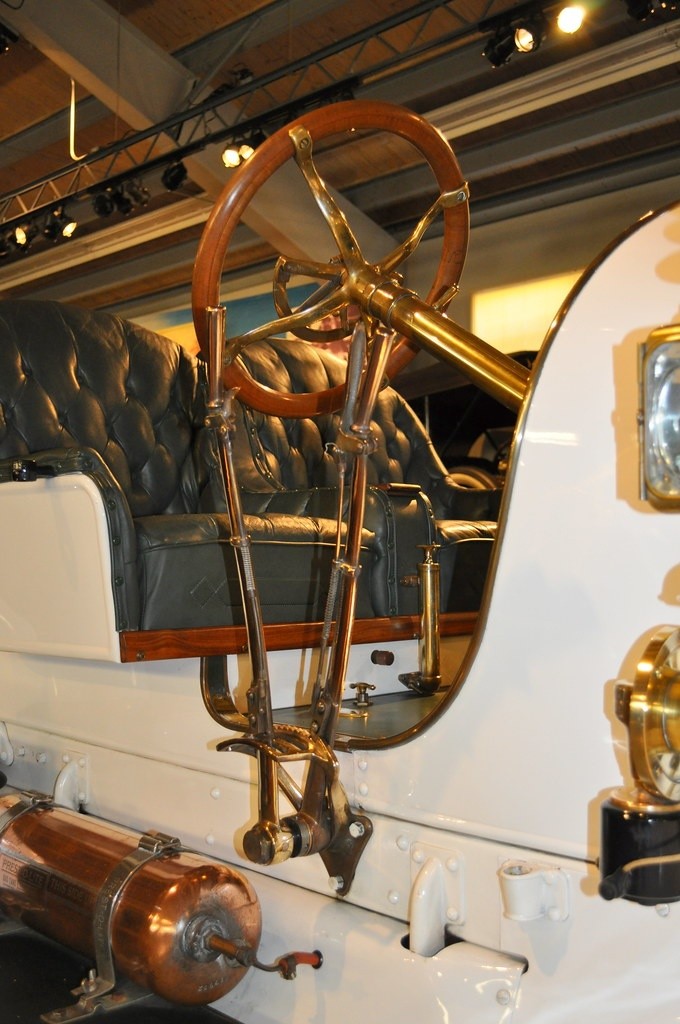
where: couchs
[0,301,506,664]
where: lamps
[482,27,515,68]
[222,134,245,168]
[513,12,550,53]
[93,182,151,217]
[162,163,188,192]
[239,132,267,160]
[0,205,78,259]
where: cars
[0,97,680,1024]
